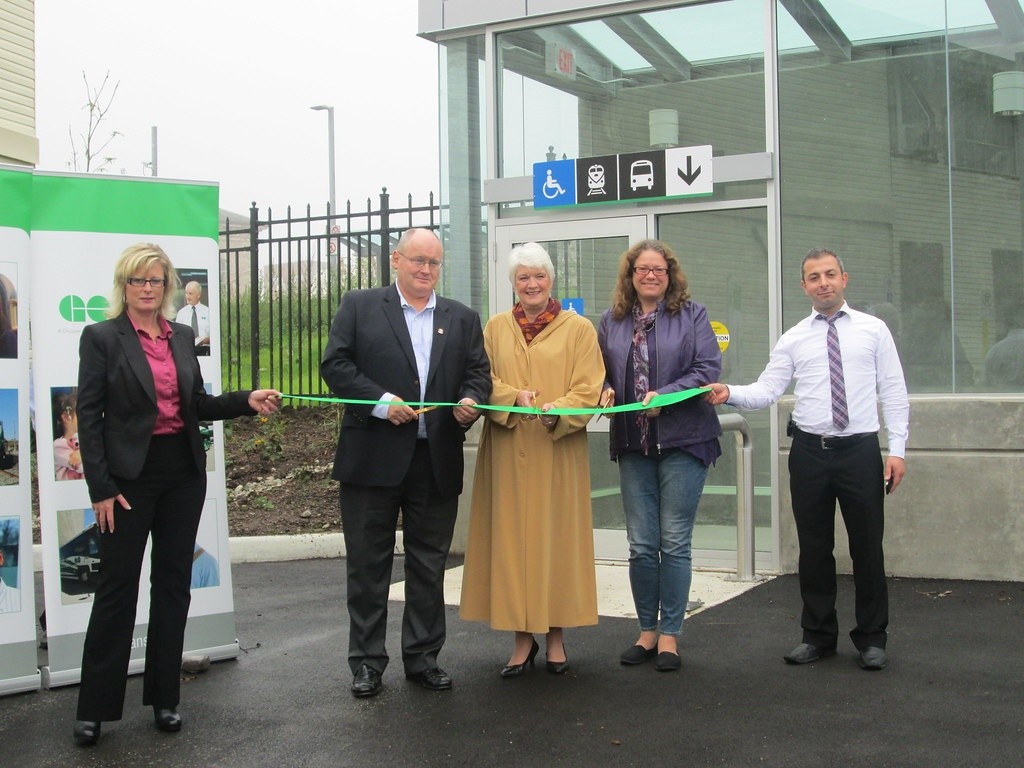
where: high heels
[500,636,539,677]
[72,719,101,745]
[153,705,182,732]
[545,641,569,674]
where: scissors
[595,388,612,422]
[405,404,437,423]
[532,391,540,420]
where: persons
[190,543,221,589]
[457,243,607,675]
[320,228,492,695]
[699,248,910,668]
[0,551,22,614]
[175,280,211,357]
[53,391,88,480]
[852,298,1024,393]
[596,239,723,669]
[72,243,283,740]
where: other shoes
[655,650,682,671]
[856,646,888,670]
[783,643,823,663]
[620,642,659,664]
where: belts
[792,426,877,450]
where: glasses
[632,266,670,276]
[126,277,167,287]
[398,252,445,269]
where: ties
[191,306,198,338]
[815,310,849,432]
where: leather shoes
[350,665,383,696]
[406,667,452,690]
